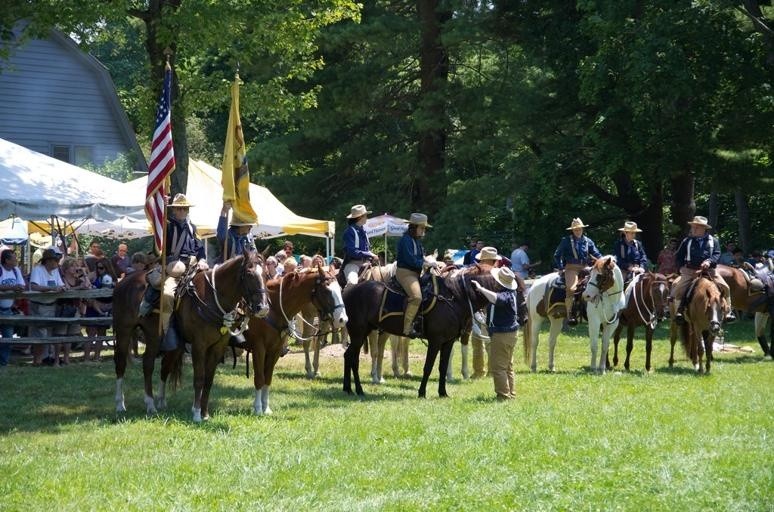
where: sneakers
[568,319,577,326]
[723,315,736,324]
[409,329,421,337]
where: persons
[340,203,543,403]
[553,215,774,363]
[0,237,147,368]
[145,192,348,349]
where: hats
[41,249,62,264]
[566,216,713,231]
[475,246,502,260]
[767,250,774,257]
[166,194,433,228]
[490,267,518,290]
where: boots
[758,335,770,356]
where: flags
[222,60,257,223]
[144,54,177,257]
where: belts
[31,300,56,306]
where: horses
[302,248,447,385]
[668,273,728,376]
[340,268,529,399]
[388,259,500,384]
[600,269,675,374]
[157,263,349,417]
[522,254,626,376]
[701,263,774,361]
[113,244,273,428]
